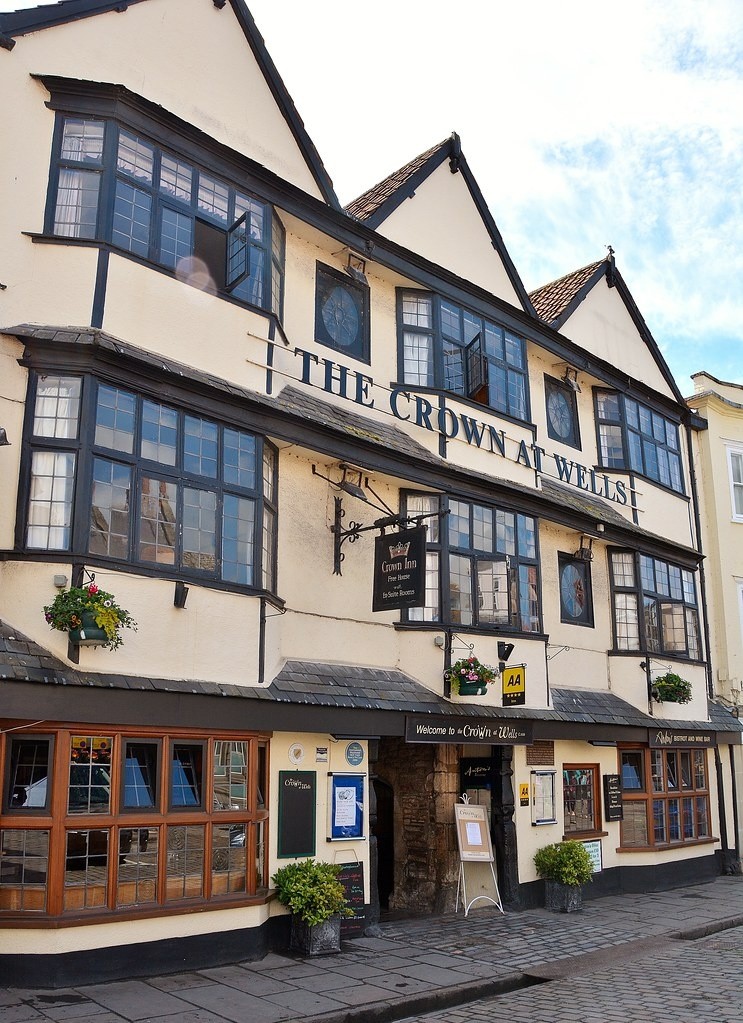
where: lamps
[574,532,599,562]
[553,363,582,394]
[332,247,373,288]
[330,460,373,501]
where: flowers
[41,583,138,651]
[652,672,694,706]
[446,654,501,696]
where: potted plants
[270,857,358,957]
[532,838,596,913]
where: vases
[662,694,680,703]
[68,609,108,645]
[458,675,487,695]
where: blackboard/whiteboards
[331,861,366,938]
[277,768,317,858]
